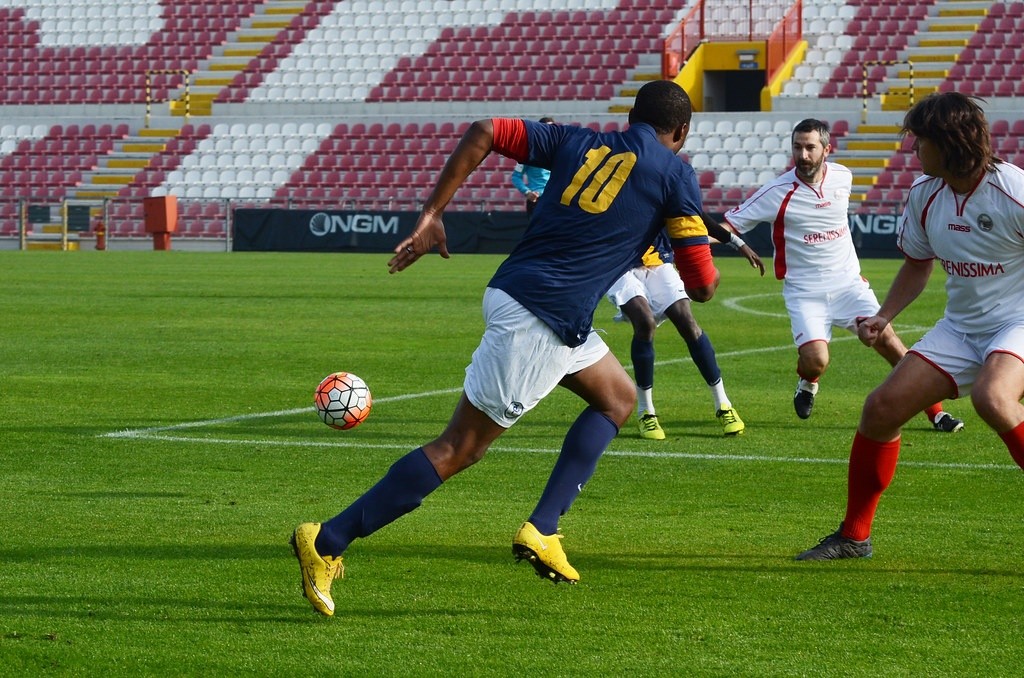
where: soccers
[312,371,374,431]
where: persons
[290,80,721,616]
[799,92,1024,561]
[708,120,964,433]
[607,212,764,439]
[511,117,554,221]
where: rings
[405,248,411,253]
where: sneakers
[794,376,819,419]
[637,410,666,440]
[796,521,872,561]
[511,522,580,585]
[289,522,345,617]
[933,411,965,433]
[715,403,745,435]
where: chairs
[1014,49,1024,64]
[939,80,955,94]
[991,136,999,154]
[965,64,986,80]
[945,64,965,81]
[0,0,934,229]
[966,2,1024,49]
[1008,120,1024,137]
[975,48,996,65]
[959,81,976,97]
[1014,80,1024,97]
[990,119,1008,137]
[956,48,976,64]
[995,49,1016,65]
[995,81,1015,97]
[985,64,1006,81]
[998,136,1019,154]
[1012,153,1024,169]
[974,80,995,97]
[1005,64,1024,80]
